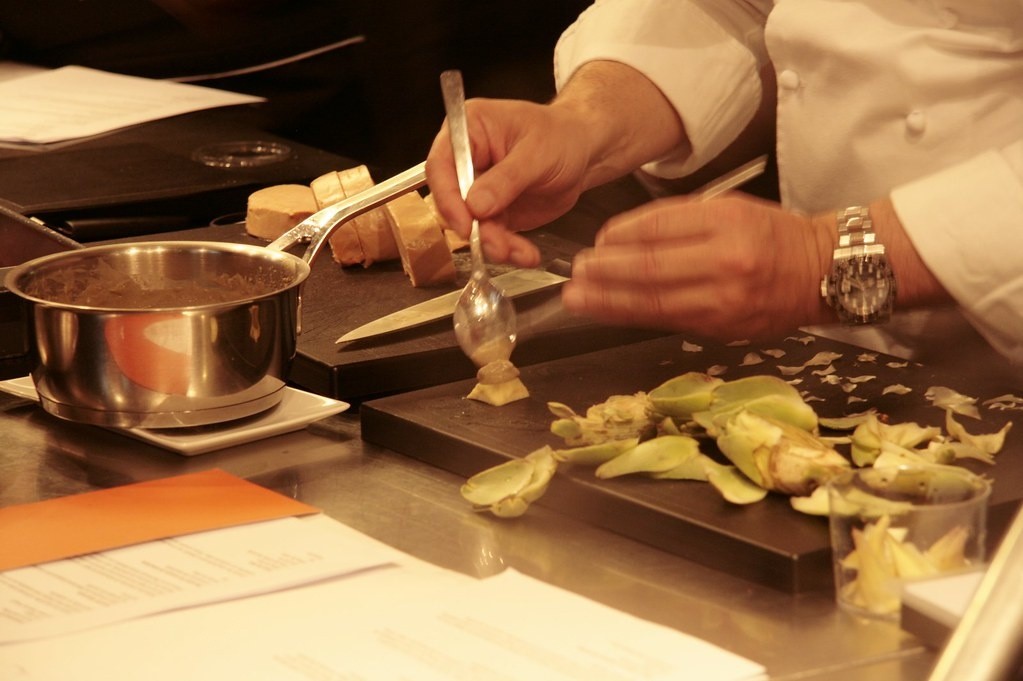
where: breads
[245,166,472,288]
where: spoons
[440,69,516,366]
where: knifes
[334,254,574,345]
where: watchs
[820,206,896,325]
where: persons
[425,0,1023,375]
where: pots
[2,161,429,430]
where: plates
[0,374,351,458]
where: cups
[829,461,991,620]
